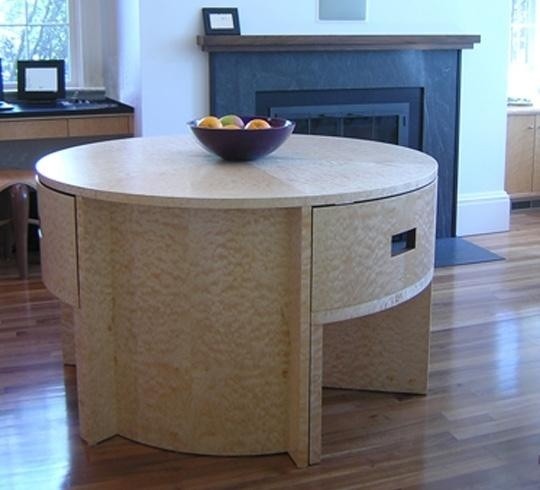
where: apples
[196,115,270,129]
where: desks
[0,98,134,142]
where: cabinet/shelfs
[505,114,540,193]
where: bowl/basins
[185,117,297,163]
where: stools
[0,168,39,281]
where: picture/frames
[17,60,65,99]
[202,7,241,35]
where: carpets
[435,235,506,268]
